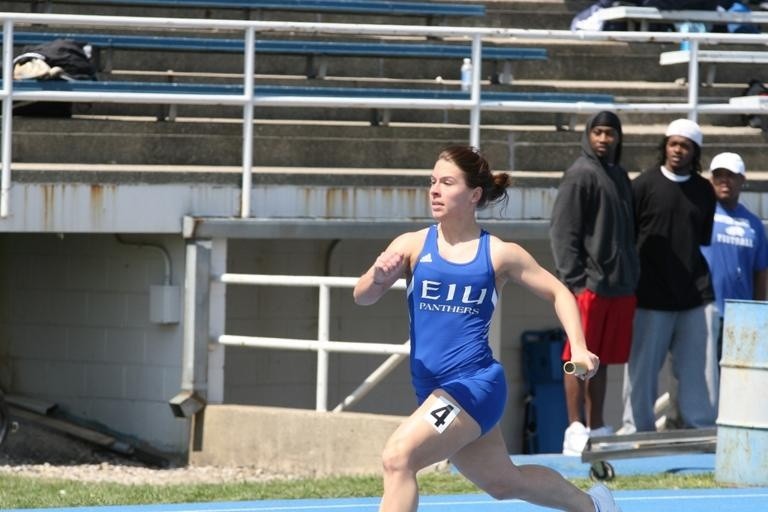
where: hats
[710,151,748,182]
[664,117,704,149]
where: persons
[352,144,625,512]
[696,149,768,377]
[617,116,716,436]
[548,109,642,454]
[568,0,768,34]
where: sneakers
[562,420,639,457]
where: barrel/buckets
[714,297,768,485]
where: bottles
[461,58,474,96]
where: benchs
[0,0,767,124]
